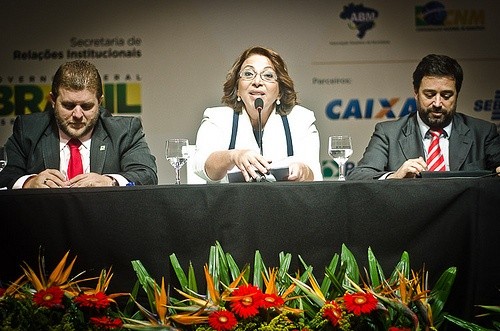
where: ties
[424,128,446,172]
[67,139,84,179]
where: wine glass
[164,138,189,184]
[327,135,353,181]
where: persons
[193,46,324,182]
[346,54,500,179]
[0,61,157,189]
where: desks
[0,175,500,331]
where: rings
[44,179,48,184]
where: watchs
[109,176,119,186]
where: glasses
[236,66,278,83]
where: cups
[0,146,8,172]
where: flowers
[0,236,457,331]
